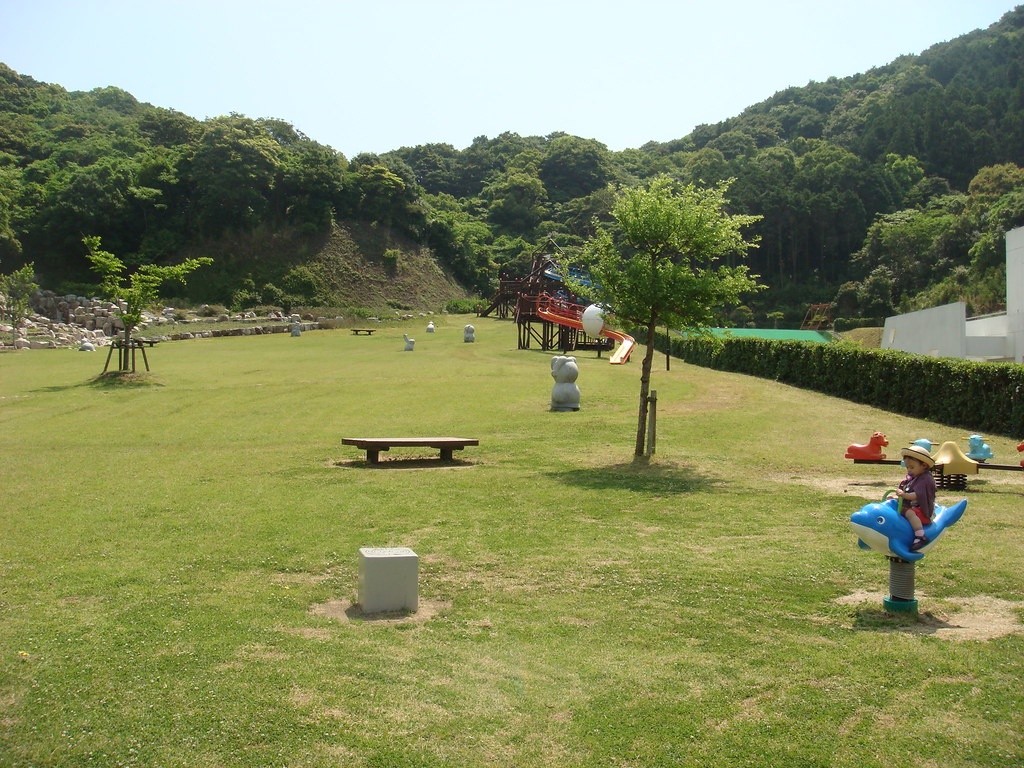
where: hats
[901,445,935,468]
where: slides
[537,307,636,365]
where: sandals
[911,534,929,551]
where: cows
[967,435,994,459]
[844,431,890,460]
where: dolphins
[850,498,968,561]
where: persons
[885,446,938,552]
[475,286,577,317]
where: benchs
[342,437,479,462]
[351,329,376,335]
[116,340,159,347]
